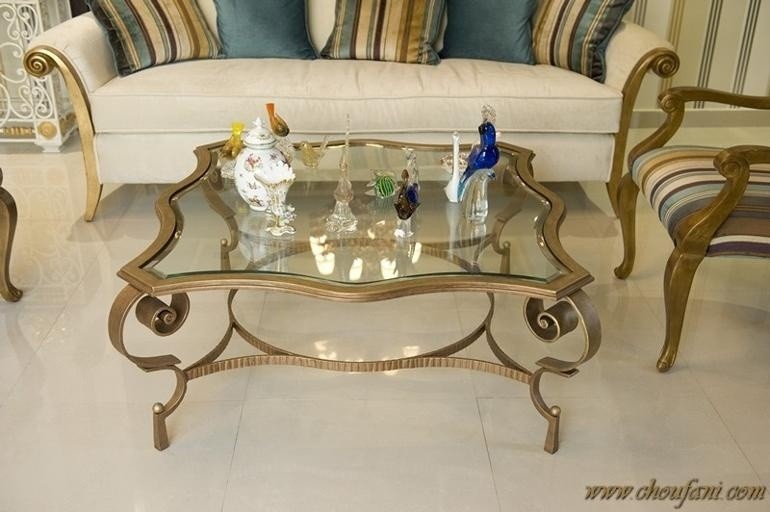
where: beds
[108,138,602,455]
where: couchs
[21,0,681,225]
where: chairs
[613,87,769,372]
